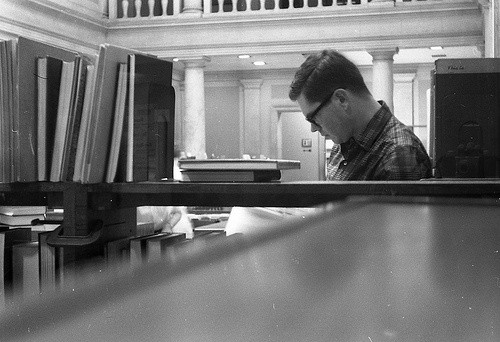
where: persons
[288,49,434,181]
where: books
[0,36,302,306]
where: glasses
[305,86,346,126]
